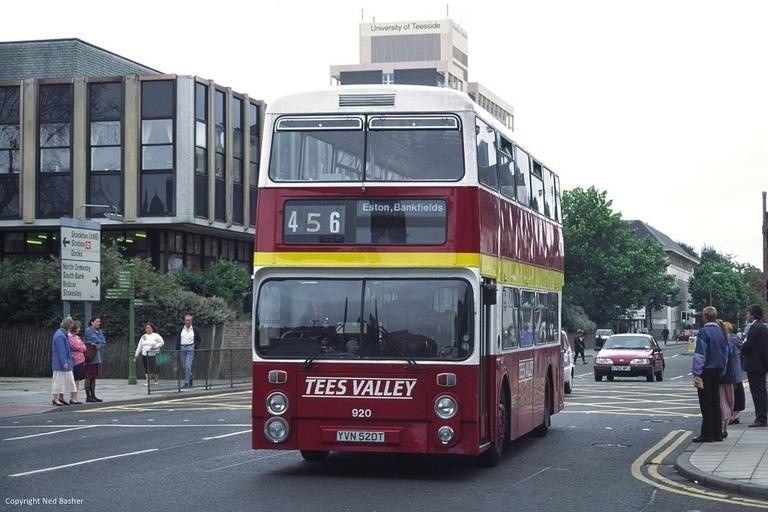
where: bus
[251,83,565,468]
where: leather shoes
[747,421,766,427]
[691,436,703,442]
[85,397,96,402]
[94,397,102,402]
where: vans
[561,330,575,393]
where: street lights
[709,270,723,306]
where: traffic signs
[105,271,130,299]
[61,260,101,300]
[134,299,145,306]
[61,227,101,261]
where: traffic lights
[681,310,687,324]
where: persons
[67,321,87,404]
[638,340,644,346]
[84,316,106,403]
[692,306,745,442]
[502,322,554,349]
[51,317,77,405]
[134,323,165,386]
[573,329,588,366]
[662,325,669,345]
[281,285,470,357]
[174,314,202,387]
[740,304,768,428]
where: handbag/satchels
[84,343,97,361]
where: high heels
[727,418,736,425]
[52,400,62,406]
[59,399,69,406]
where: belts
[180,344,192,346]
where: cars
[594,329,615,346]
[675,329,699,341]
[594,332,666,382]
